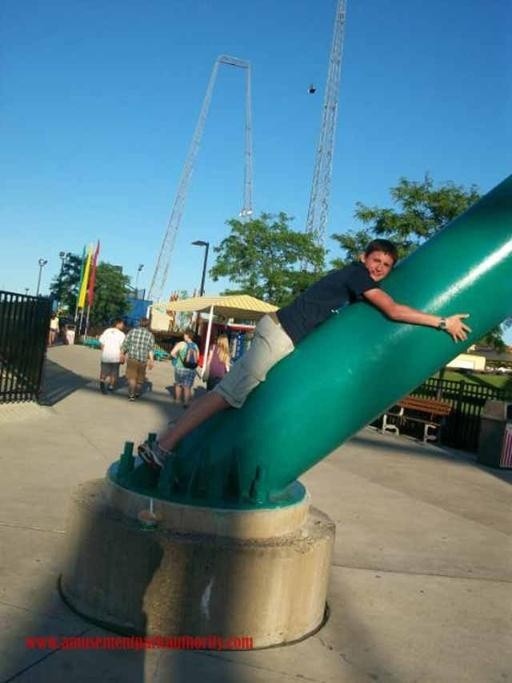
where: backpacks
[179,341,198,369]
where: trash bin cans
[64,324,76,345]
[476,399,512,469]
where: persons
[47,312,58,346]
[120,316,157,401]
[203,333,232,392]
[97,316,128,394]
[136,236,472,471]
[169,328,201,410]
[53,312,60,343]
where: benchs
[381,396,453,446]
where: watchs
[436,316,447,331]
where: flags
[87,240,101,306]
[77,243,93,309]
[75,244,86,306]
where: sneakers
[100,381,115,394]
[138,433,175,476]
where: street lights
[192,239,209,342]
[56,251,72,316]
